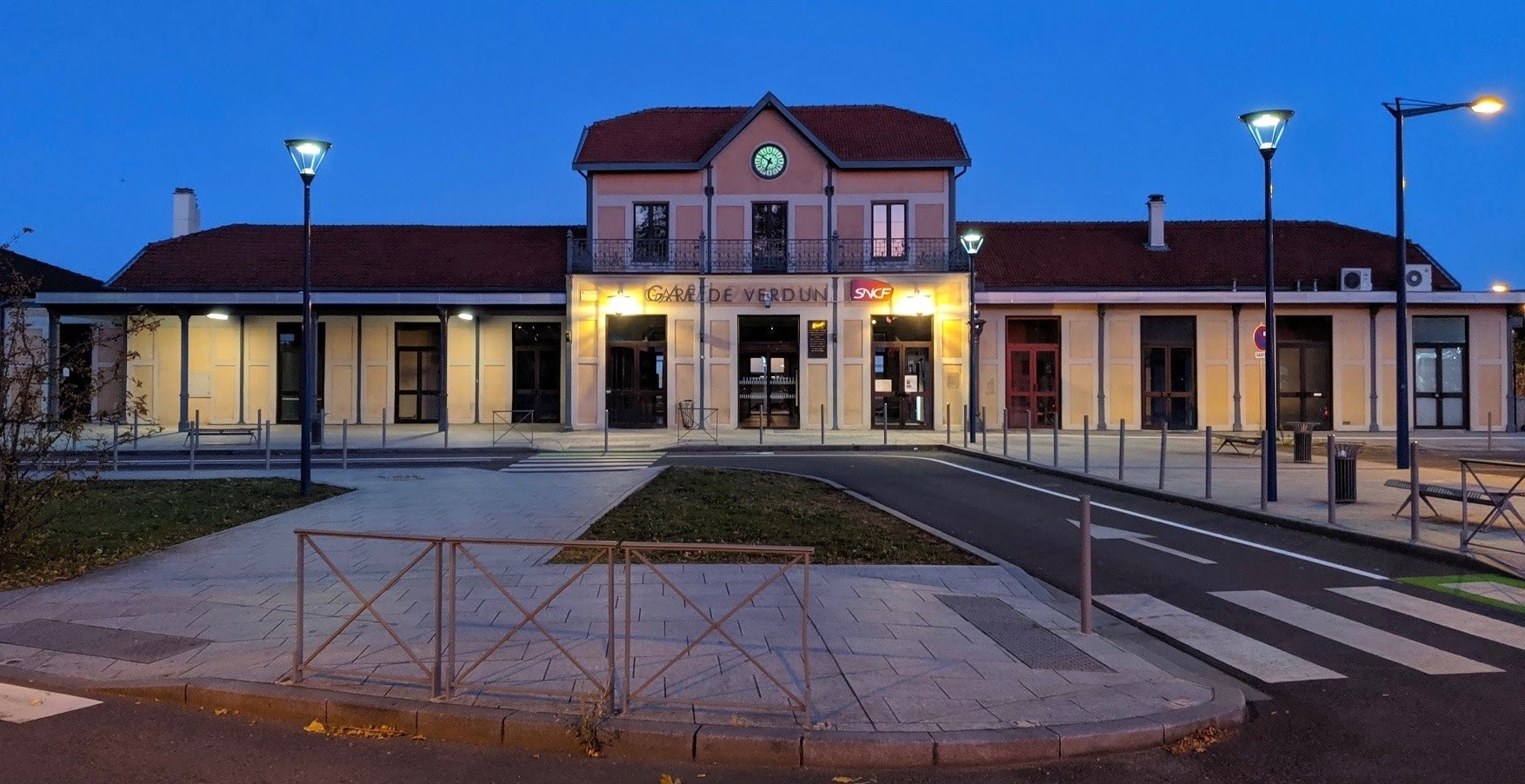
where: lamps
[207,307,229,320]
[458,312,473,320]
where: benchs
[1211,432,1281,458]
[183,428,261,445]
[1383,479,1525,533]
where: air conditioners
[1405,263,1432,292]
[1341,267,1373,291]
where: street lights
[1378,95,1507,469]
[1239,108,1299,501]
[959,228,989,445]
[280,138,334,497]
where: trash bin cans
[1334,441,1366,504]
[1287,421,1321,463]
[678,400,696,428]
[311,413,328,444]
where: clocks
[749,141,788,182]
